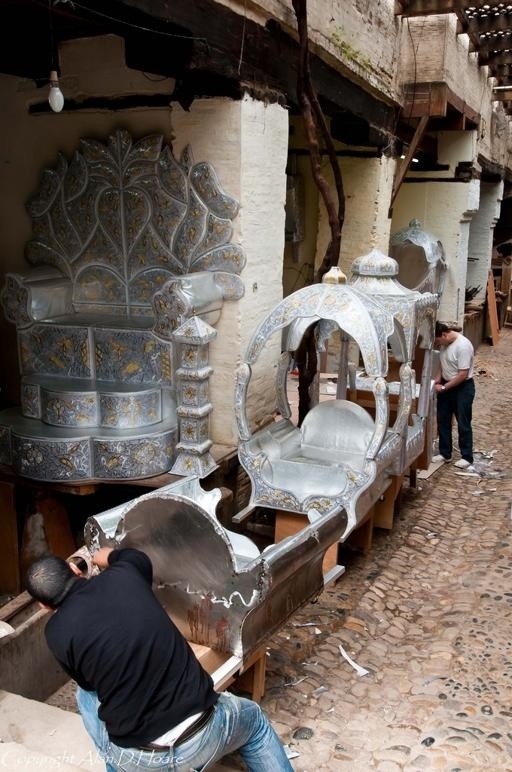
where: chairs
[82,476,349,704]
[231,245,447,591]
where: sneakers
[431,455,453,463]
[453,458,471,469]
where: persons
[433,322,476,468]
[24,544,294,771]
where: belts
[137,706,216,752]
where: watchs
[441,385,446,392]
